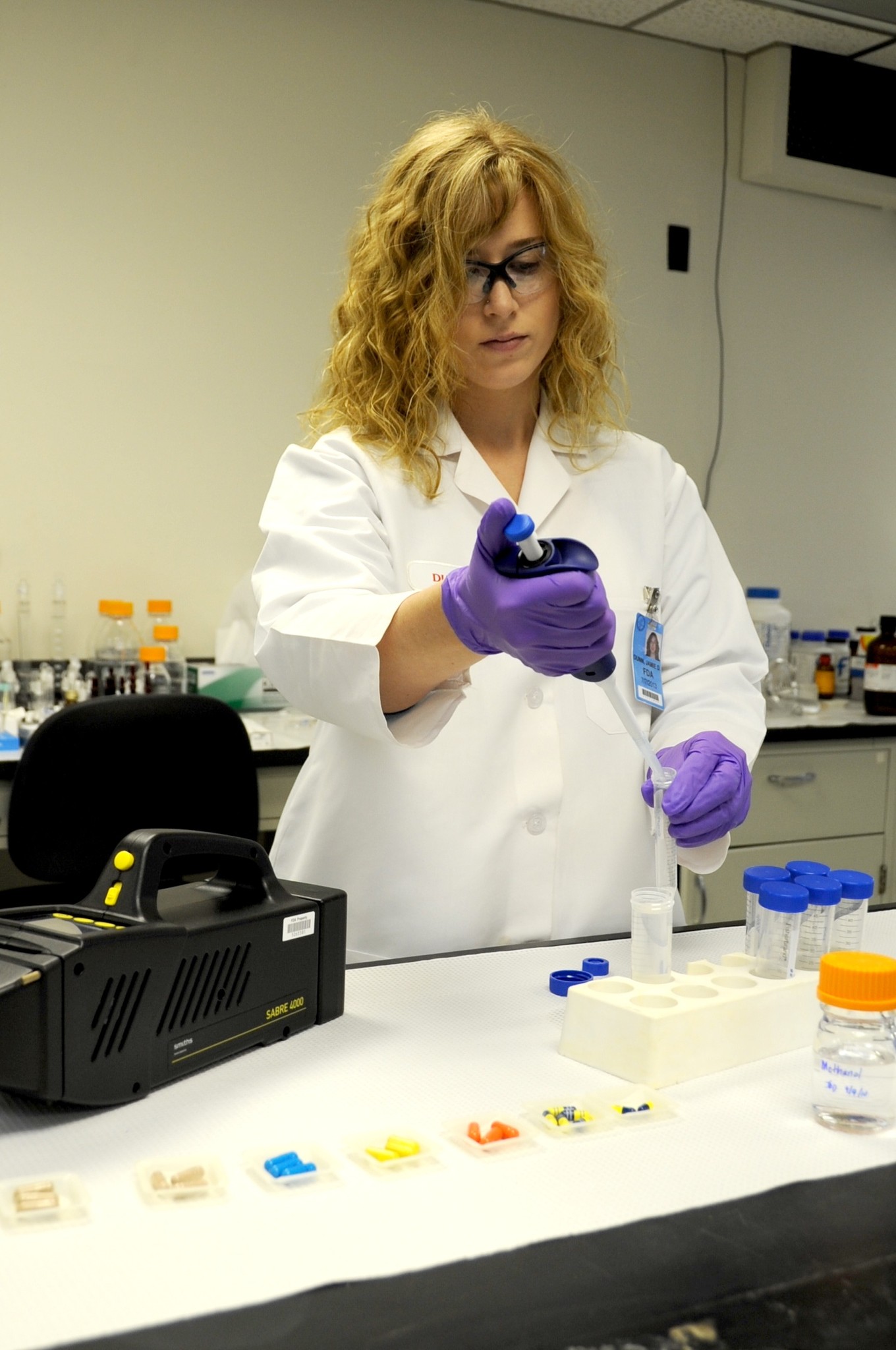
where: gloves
[440,499,618,677]
[637,731,754,849]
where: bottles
[746,586,896,717]
[812,950,896,1134]
[93,599,189,694]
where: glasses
[457,238,563,306]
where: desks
[0,900,896,1349]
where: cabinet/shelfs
[677,734,896,928]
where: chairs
[6,693,261,900]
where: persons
[251,114,768,963]
[646,632,659,661]
